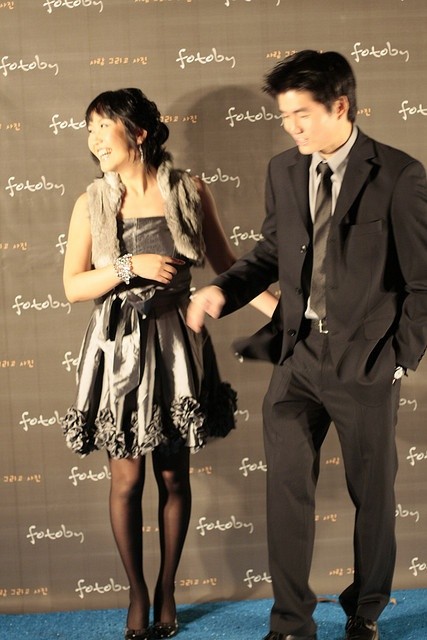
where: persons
[62,88,279,640]
[185,49,427,640]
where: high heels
[153,603,179,640]
[124,603,151,640]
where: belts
[306,319,328,334]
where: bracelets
[112,254,137,285]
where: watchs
[393,365,405,379]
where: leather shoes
[345,614,379,639]
[263,630,318,639]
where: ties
[309,161,334,322]
